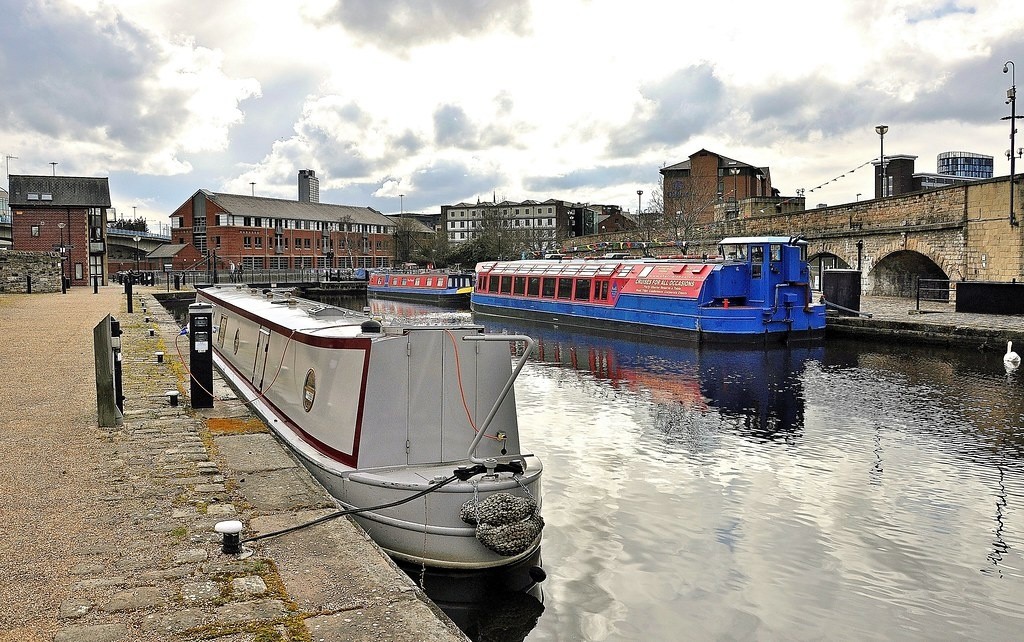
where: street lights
[49,162,57,176]
[1002,61,1024,226]
[133,206,137,221]
[876,125,888,199]
[133,236,142,283]
[250,182,256,197]
[637,191,644,226]
[56,223,66,294]
[399,195,404,215]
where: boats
[471,237,827,343]
[367,272,473,295]
[190,284,547,614]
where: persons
[229,270,233,283]
[237,262,243,282]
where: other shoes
[241,281,243,283]
[238,281,239,282]
[231,281,233,283]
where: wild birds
[1003,341,1021,362]
